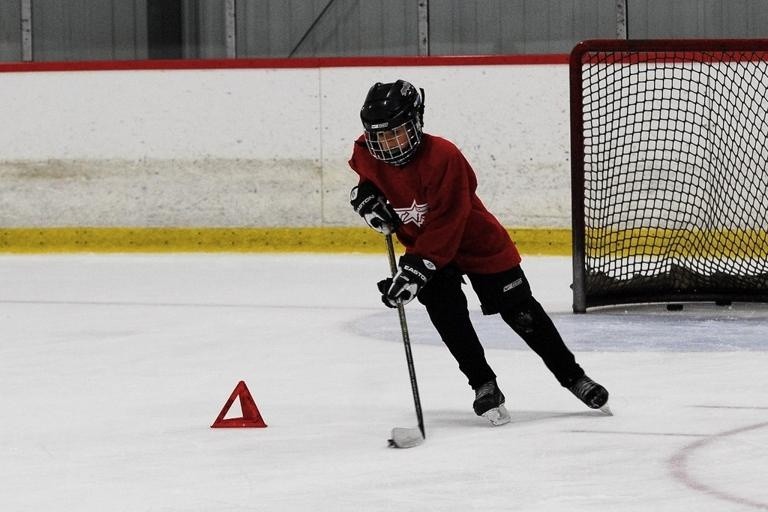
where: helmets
[361,80,425,168]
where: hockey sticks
[385,233,425,447]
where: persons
[345,79,609,418]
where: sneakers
[569,373,608,409]
[473,380,506,416]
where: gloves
[349,187,400,235]
[378,253,437,308]
